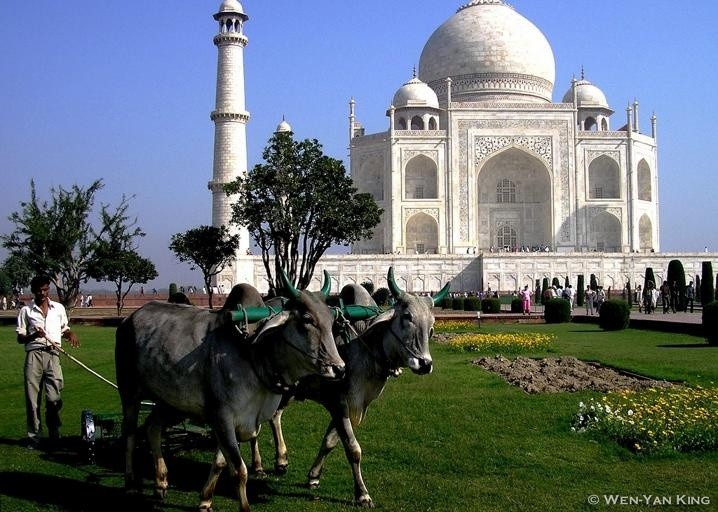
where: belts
[37,346,55,351]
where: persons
[80,291,94,307]
[704,246,707,252]
[447,290,499,300]
[512,290,517,296]
[519,285,531,316]
[152,287,158,296]
[1,294,21,311]
[543,285,575,314]
[140,286,144,296]
[466,245,549,254]
[488,282,491,292]
[634,281,696,315]
[15,276,81,450]
[583,283,605,315]
[179,284,229,294]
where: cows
[112,264,453,511]
